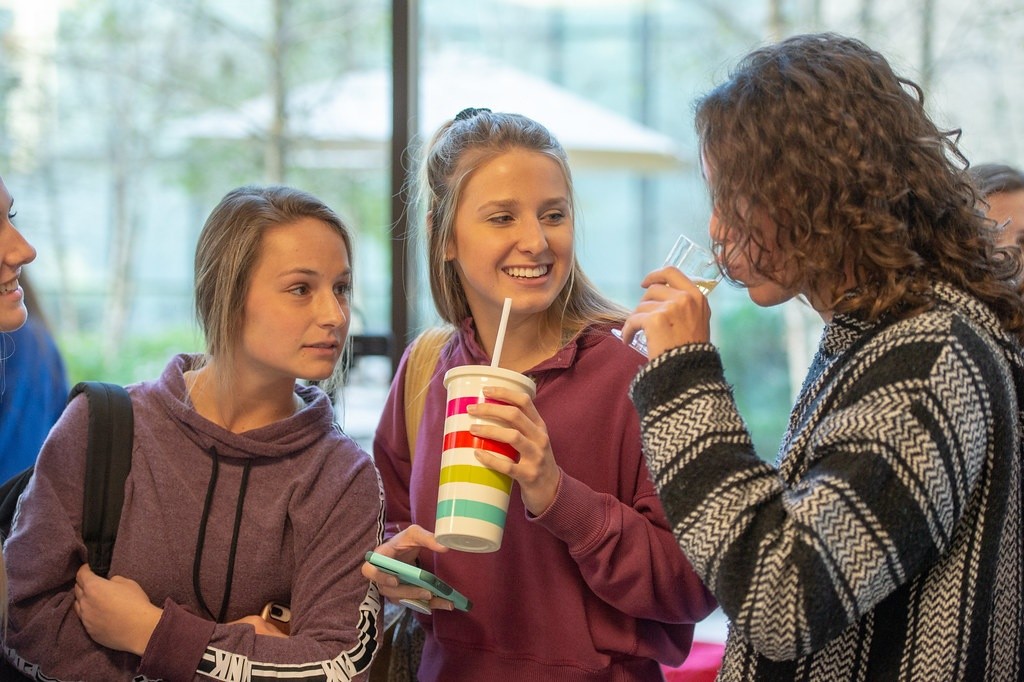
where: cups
[434,363,536,555]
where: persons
[619,29,1024,682]
[0,175,72,487]
[362,106,720,682]
[955,163,1024,306]
[0,187,386,682]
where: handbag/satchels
[367,327,458,682]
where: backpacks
[0,382,134,579]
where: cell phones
[363,551,473,614]
[261,601,291,638]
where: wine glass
[611,232,728,357]
